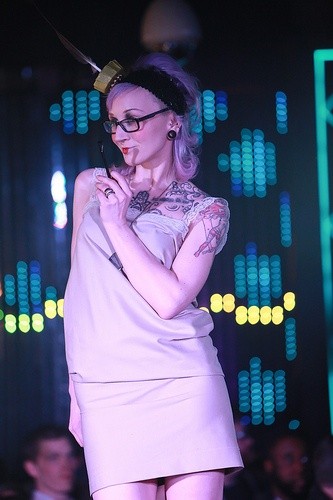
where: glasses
[102,106,173,134]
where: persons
[0,410,332,499]
[61,55,243,500]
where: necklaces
[127,180,176,228]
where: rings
[104,188,115,198]
